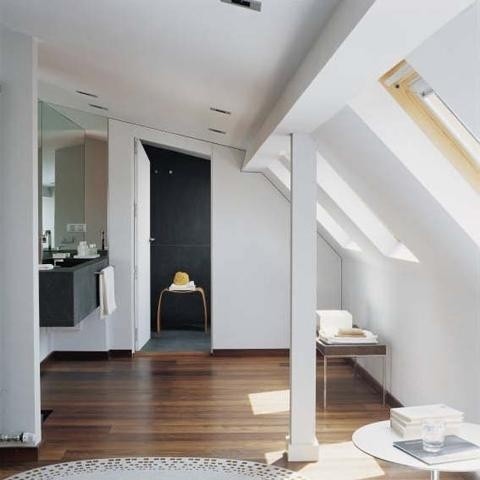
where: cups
[422,420,447,453]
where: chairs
[158,260,207,334]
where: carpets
[6,457,313,480]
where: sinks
[42,257,93,268]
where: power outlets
[68,224,86,233]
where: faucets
[44,230,51,250]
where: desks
[316,337,388,409]
[352,418,480,480]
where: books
[320,331,380,347]
[388,400,465,439]
[391,432,480,468]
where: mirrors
[39,101,85,252]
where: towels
[99,267,118,318]
[39,264,54,271]
